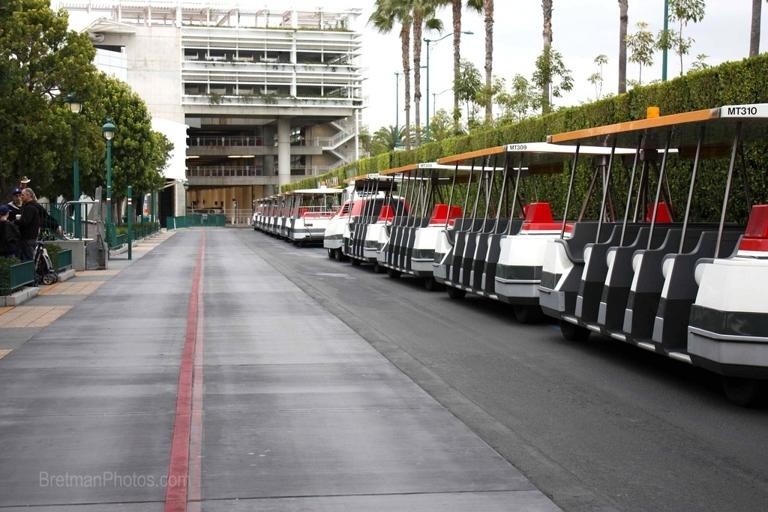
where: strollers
[32,240,58,287]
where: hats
[16,175,31,184]
[0,205,12,212]
[13,188,21,194]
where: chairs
[646,201,673,224]
[519,201,555,222]
[377,206,395,222]
[734,203,767,259]
[428,203,463,225]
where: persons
[0,176,67,261]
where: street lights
[102,119,115,249]
[66,88,82,236]
[394,31,474,140]
